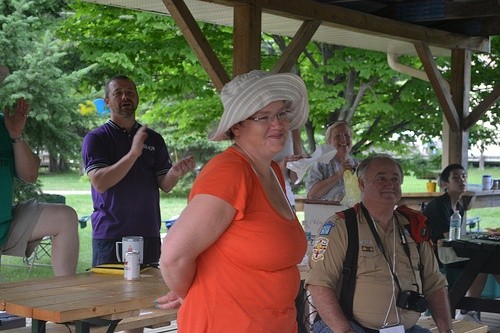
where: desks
[0,265,177,333]
[436,227,500,318]
[399,188,500,217]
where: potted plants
[425,174,436,192]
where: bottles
[449,210,462,241]
[301,220,312,257]
[482,175,492,190]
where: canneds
[482,175,492,190]
[124,251,140,281]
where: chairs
[23,194,70,265]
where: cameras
[396,290,427,313]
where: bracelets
[8,132,24,143]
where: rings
[24,114,28,117]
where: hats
[206,69,309,140]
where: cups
[493,179,500,190]
[115,236,144,264]
[426,183,436,192]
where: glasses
[247,109,292,124]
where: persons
[0,97,79,276]
[305,154,452,333]
[154,70,309,333]
[272,129,302,214]
[306,120,363,202]
[82,75,195,268]
[423,163,486,324]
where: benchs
[412,312,489,333]
[0,307,180,333]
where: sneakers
[455,310,492,332]
